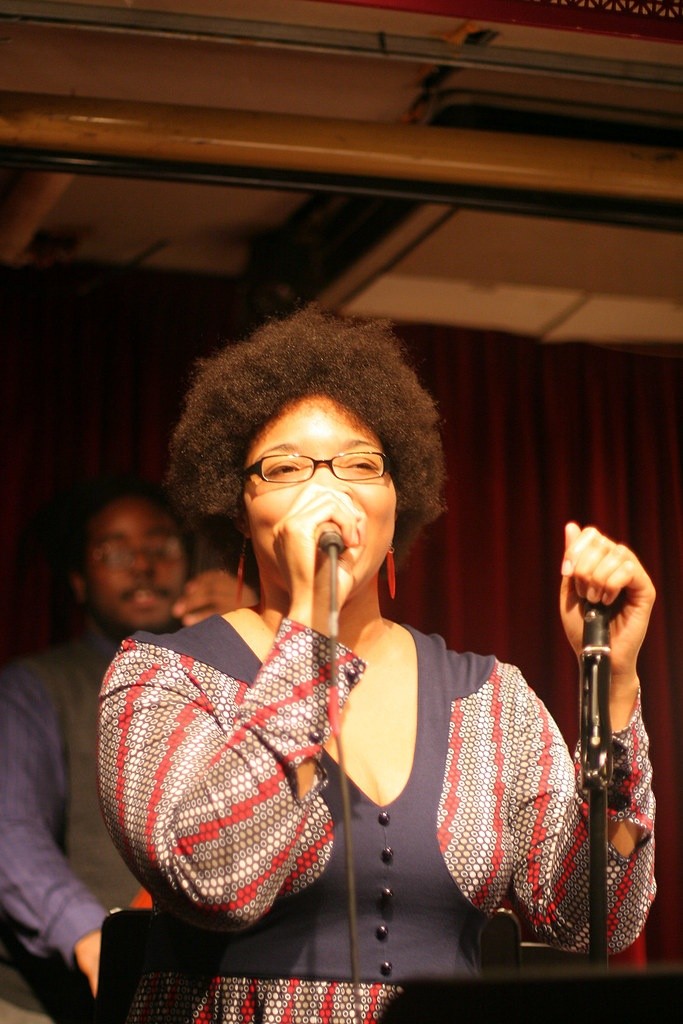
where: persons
[95,303,657,1024]
[0,470,260,1000]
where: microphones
[317,521,345,553]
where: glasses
[245,452,389,483]
[84,532,186,572]
